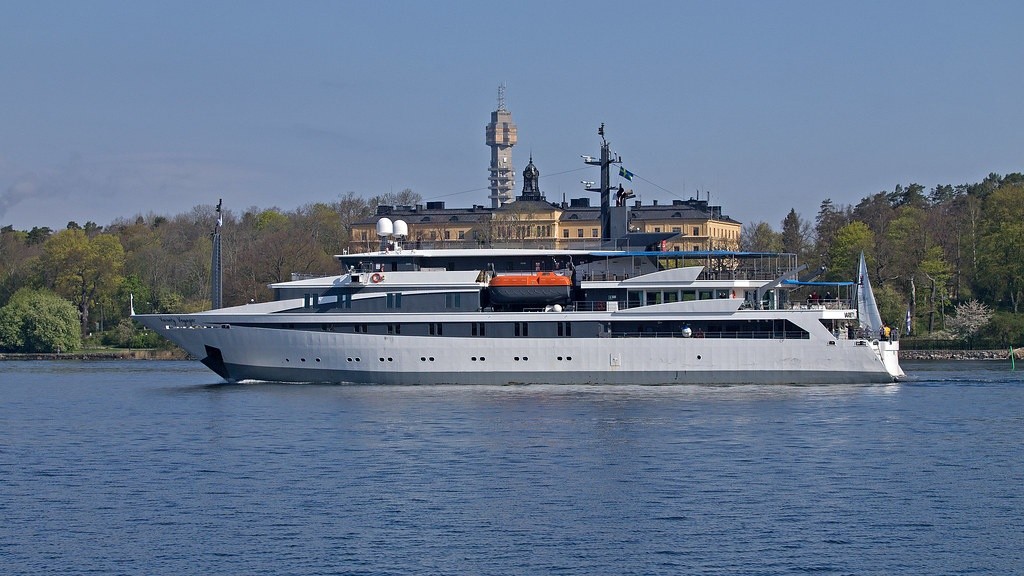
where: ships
[128,121,905,384]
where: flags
[906,306,910,335]
[618,167,633,181]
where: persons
[845,323,854,339]
[475,235,494,249]
[813,291,819,301]
[865,326,871,338]
[806,294,813,303]
[825,292,832,302]
[719,293,726,299]
[880,324,890,340]
[625,274,629,279]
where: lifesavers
[372,273,381,283]
